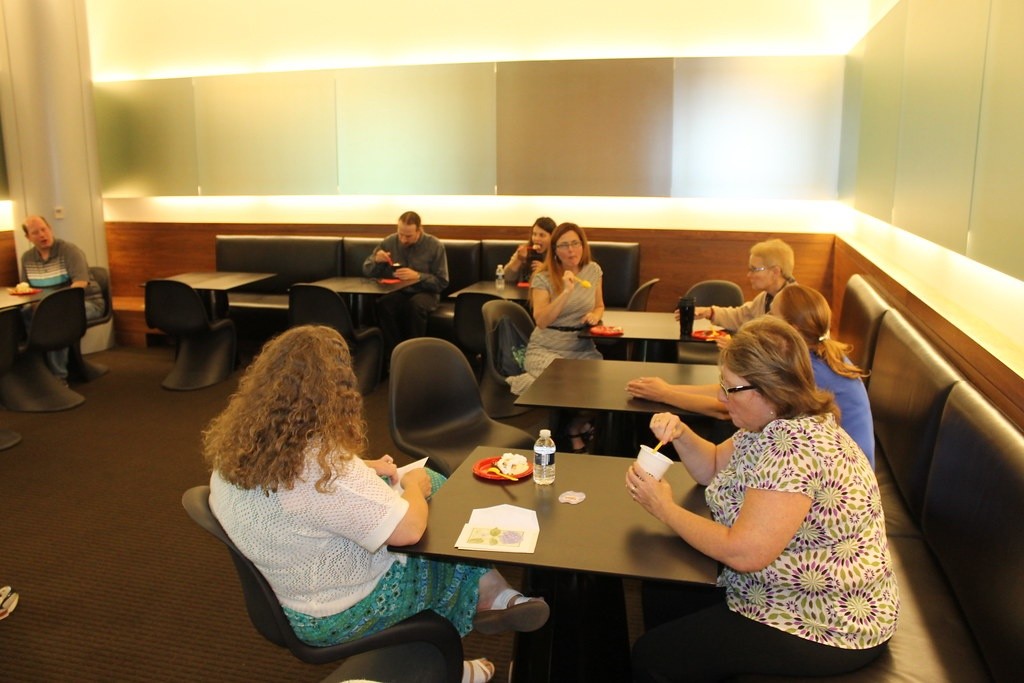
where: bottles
[495,265,505,289]
[533,429,556,485]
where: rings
[654,419,660,423]
[632,494,638,501]
[631,486,637,494]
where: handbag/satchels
[494,316,529,375]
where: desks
[161,272,278,323]
[0,285,62,309]
[577,309,727,363]
[512,358,723,458]
[386,445,720,683]
[446,282,532,301]
[287,275,420,334]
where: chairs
[144,279,549,683]
[595,278,661,363]
[675,280,744,364]
[0,266,113,452]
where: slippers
[461,658,495,683]
[473,588,549,634]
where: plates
[694,330,727,339]
[472,456,533,479]
[10,289,43,295]
[591,326,623,336]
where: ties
[764,293,773,313]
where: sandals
[569,432,589,454]
[580,422,596,444]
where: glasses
[555,241,581,251]
[719,375,757,399]
[748,266,770,274]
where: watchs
[417,272,421,281]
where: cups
[391,264,402,273]
[636,444,674,481]
[678,296,696,334]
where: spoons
[575,277,592,289]
[487,467,518,481]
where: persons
[675,239,830,352]
[21,214,105,388]
[209,325,551,683]
[362,211,449,383]
[503,217,556,282]
[625,314,901,683]
[625,283,876,475]
[522,223,605,378]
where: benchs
[213,234,640,353]
[833,272,1023,683]
